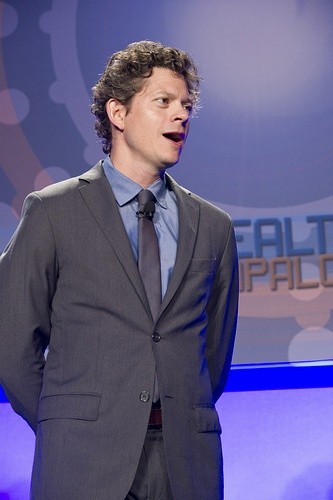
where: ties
[136,189,163,405]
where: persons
[0,40,240,500]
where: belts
[147,408,163,427]
